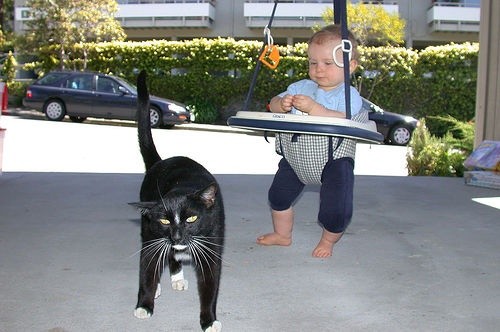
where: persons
[256,25,362,258]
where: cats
[128,68,226,332]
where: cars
[23,71,190,129]
[266,95,420,147]
